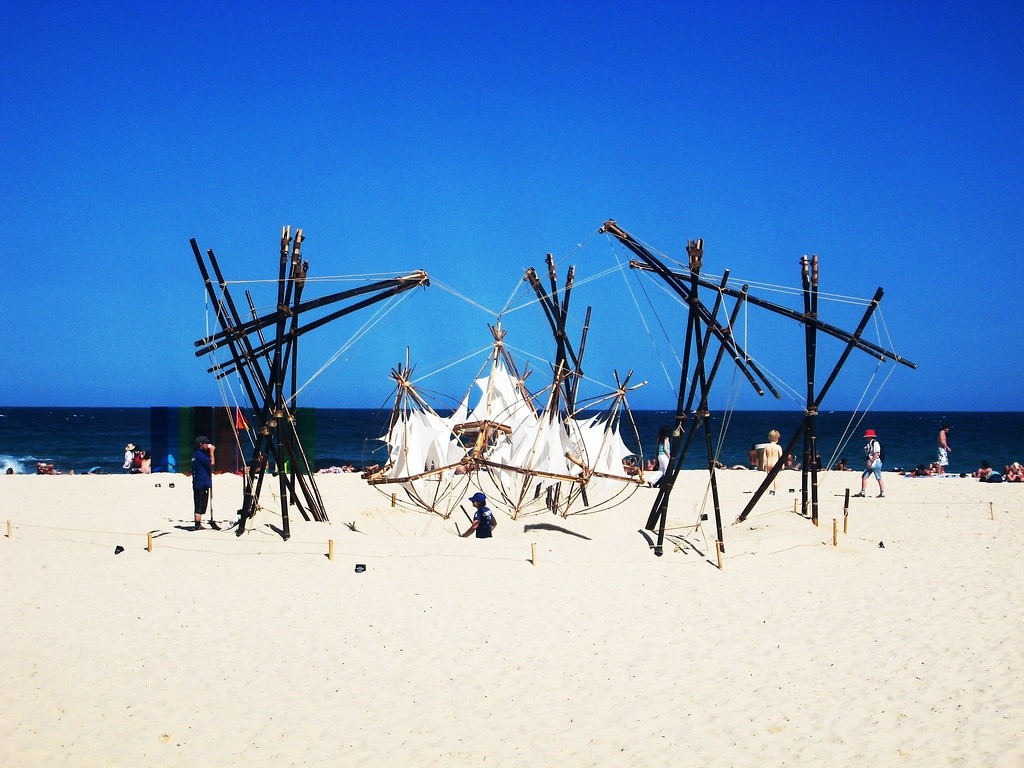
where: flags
[235,407,249,429]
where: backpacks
[871,439,885,462]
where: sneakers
[855,492,865,497]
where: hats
[468,492,486,501]
[195,436,210,445]
[125,444,135,451]
[863,430,877,438]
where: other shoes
[876,494,885,498]
[194,524,205,530]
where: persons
[36,461,111,475]
[762,430,785,490]
[648,425,675,489]
[972,459,1024,483]
[853,429,886,499]
[254,455,270,474]
[460,492,498,538]
[786,450,853,472]
[122,443,151,474]
[908,423,952,478]
[191,435,216,530]
[716,443,760,470]
[342,460,379,473]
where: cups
[208,443,215,449]
